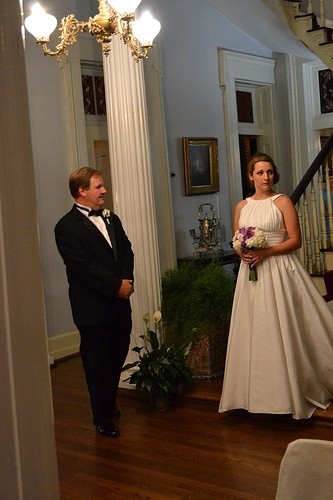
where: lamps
[25,0,161,63]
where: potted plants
[161,257,236,380]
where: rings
[250,259,252,261]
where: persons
[219,153,333,420]
[54,165,134,438]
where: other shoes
[96,421,120,438]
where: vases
[150,389,169,412]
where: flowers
[121,310,200,393]
[232,227,268,281]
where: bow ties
[87,208,104,217]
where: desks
[177,250,241,279]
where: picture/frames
[182,136,220,196]
